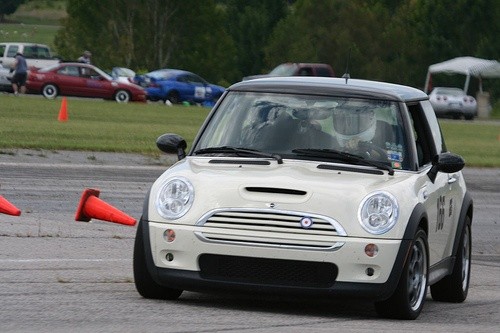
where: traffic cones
[0,194,22,217]
[57,95,68,122]
[74,188,137,227]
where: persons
[7,53,28,96]
[76,51,94,77]
[330,100,392,162]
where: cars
[130,71,478,322]
[25,59,146,104]
[427,86,478,121]
[134,68,226,108]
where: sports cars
[105,66,136,83]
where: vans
[0,42,54,92]
[267,62,335,78]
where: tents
[424,56,500,98]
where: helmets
[332,100,377,150]
